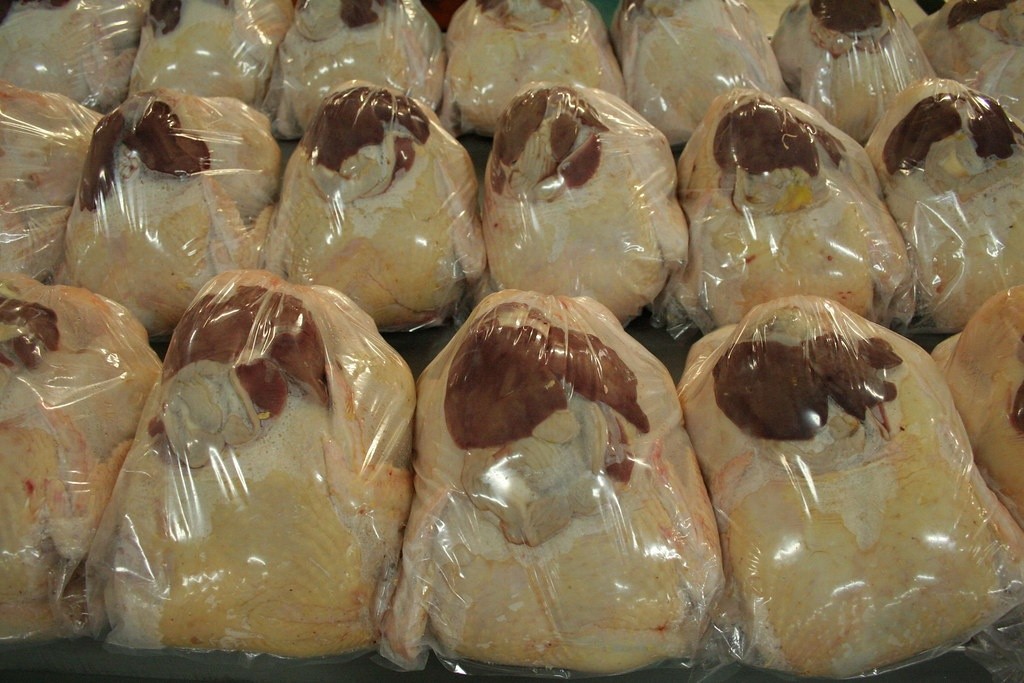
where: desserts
[0,1,1024,683]
